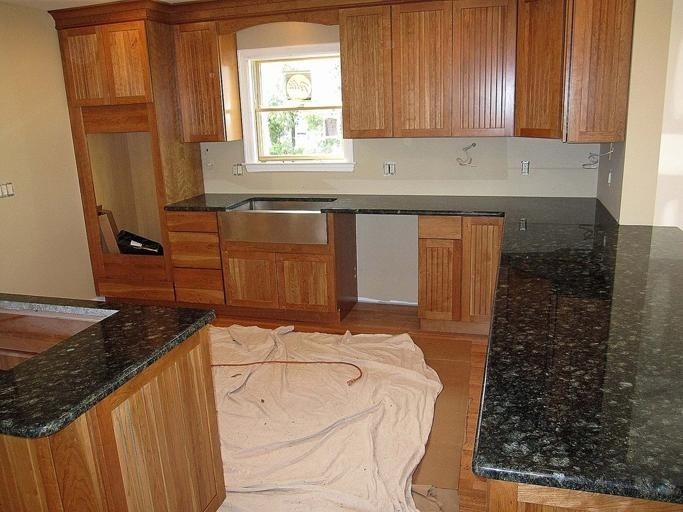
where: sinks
[220,200,332,246]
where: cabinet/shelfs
[220,240,358,323]
[46,0,173,108]
[339,0,635,145]
[165,211,225,305]
[68,103,205,307]
[461,216,502,323]
[172,0,243,143]
[418,215,462,321]
